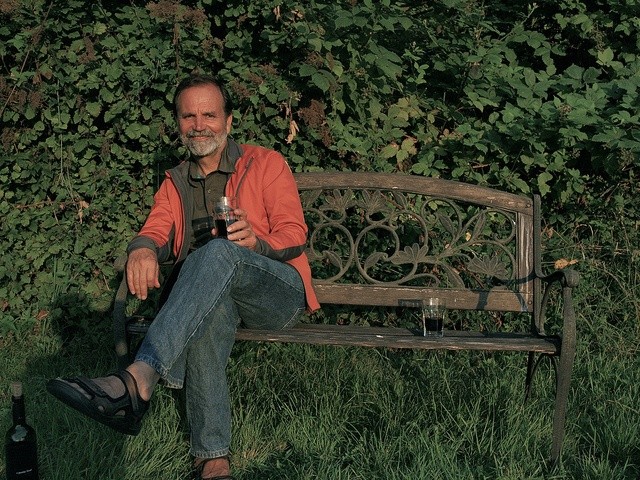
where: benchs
[112,173,578,471]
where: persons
[47,74,320,480]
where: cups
[213,196,238,239]
[423,297,445,338]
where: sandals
[195,456,234,480]
[47,369,150,436]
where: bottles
[5,383,38,480]
